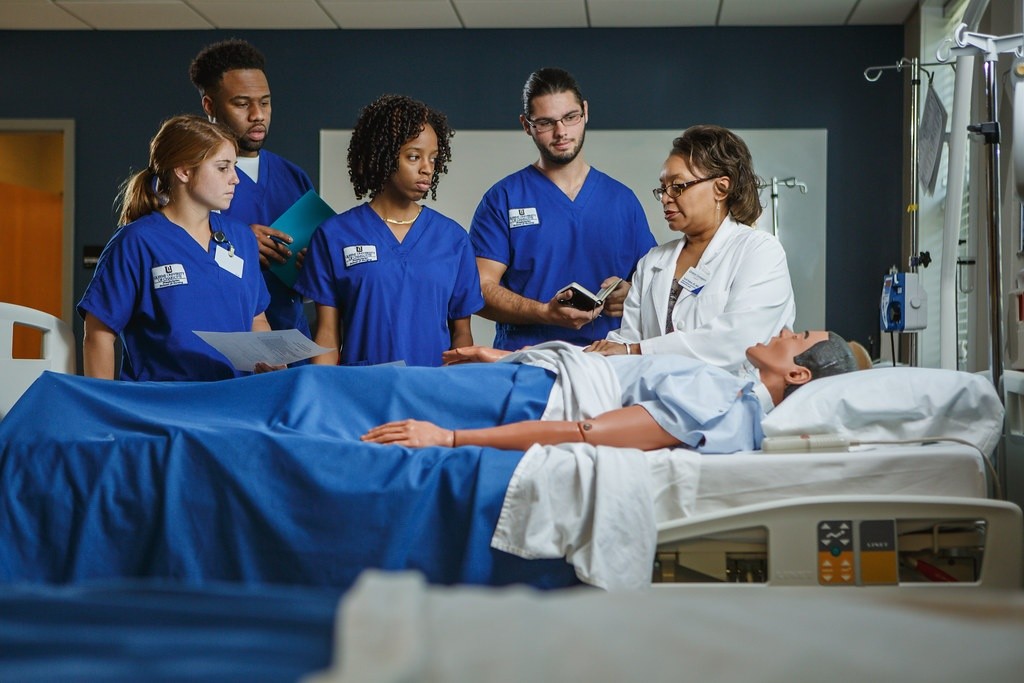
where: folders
[258,188,344,289]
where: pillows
[761,364,1008,457]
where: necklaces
[370,202,421,224]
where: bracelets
[623,342,631,356]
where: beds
[0,305,1024,683]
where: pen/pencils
[267,234,290,249]
[558,298,603,319]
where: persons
[76,114,289,383]
[298,95,486,368]
[467,67,659,351]
[361,325,861,453]
[442,124,798,379]
[188,36,324,371]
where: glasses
[524,110,585,133]
[653,172,731,201]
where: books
[555,278,623,312]
